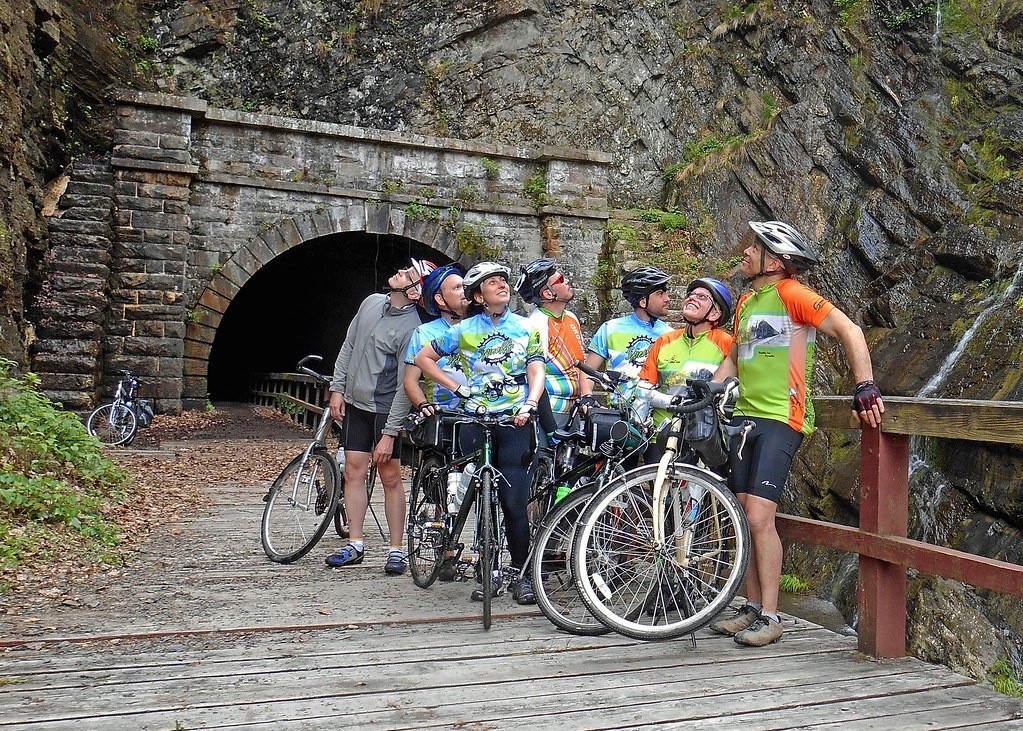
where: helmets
[749,219,818,276]
[422,266,462,316]
[513,258,557,304]
[411,256,438,286]
[461,262,511,302]
[687,278,732,327]
[620,265,674,307]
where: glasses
[401,266,420,293]
[542,273,564,297]
[684,292,722,316]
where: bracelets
[528,399,537,403]
[417,401,429,408]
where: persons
[413,261,545,605]
[325,258,437,575]
[579,266,675,584]
[403,266,481,583]
[635,278,733,616]
[513,258,587,525]
[709,221,884,646]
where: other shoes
[645,579,680,615]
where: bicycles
[87,370,150,446]
[260,353,752,647]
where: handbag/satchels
[135,399,155,429]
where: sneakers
[471,577,505,601]
[439,558,458,581]
[734,610,785,646]
[325,544,364,566]
[513,576,536,605]
[385,549,409,576]
[710,604,759,635]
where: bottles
[453,462,476,507]
[570,476,591,493]
[447,464,462,513]
[680,480,691,516]
[335,447,345,472]
[553,482,572,506]
